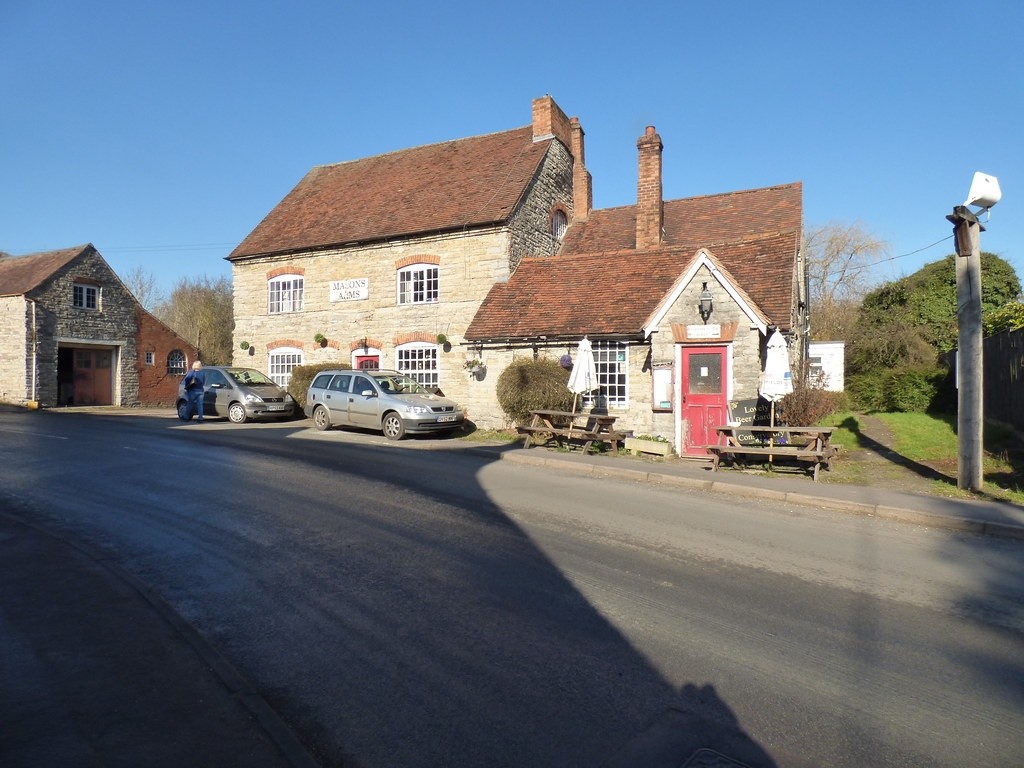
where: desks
[712,426,838,482]
[523,410,619,455]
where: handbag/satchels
[184,376,196,391]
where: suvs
[304,368,464,441]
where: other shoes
[181,419,188,422]
[197,421,202,423]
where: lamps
[699,281,713,319]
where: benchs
[514,424,626,441]
[707,441,840,461]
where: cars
[175,365,296,424]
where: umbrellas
[567,336,600,439]
[760,326,794,473]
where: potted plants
[463,360,483,373]
[624,434,671,456]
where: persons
[182,361,205,423]
